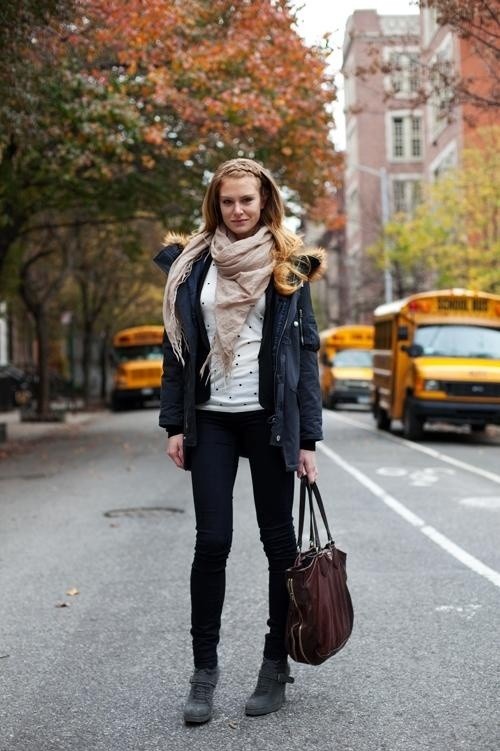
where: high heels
[184,664,218,724]
[245,657,293,715]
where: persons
[153,158,328,723]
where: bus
[110,324,165,413]
[318,323,375,410]
[369,287,500,440]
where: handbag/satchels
[284,479,354,667]
